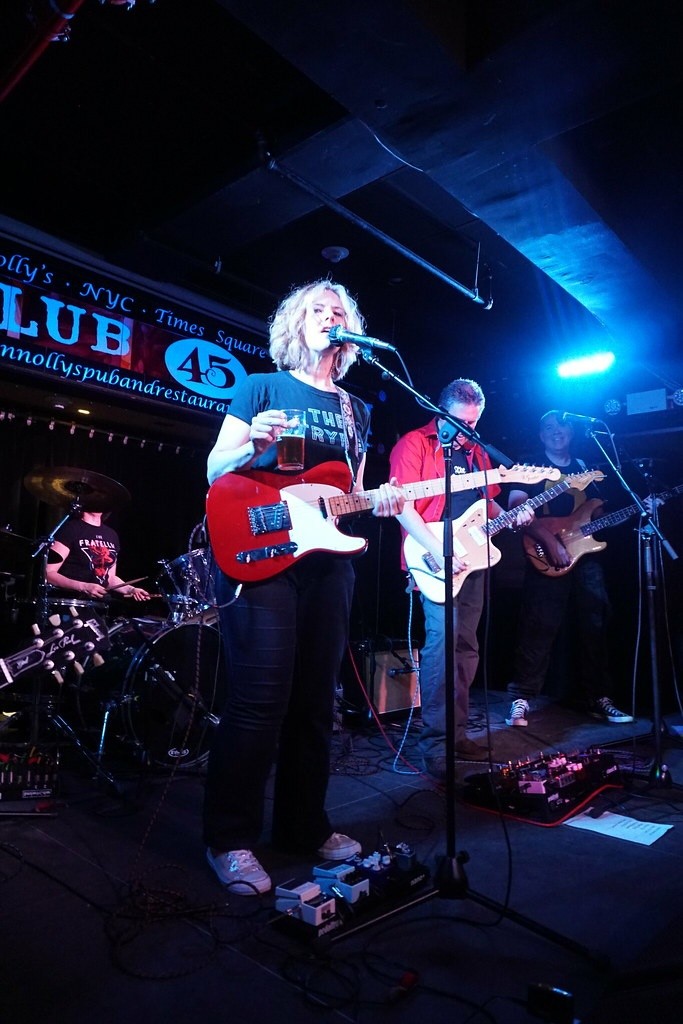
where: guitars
[1,607,111,695]
[205,461,563,582]
[519,484,682,577]
[403,467,608,604]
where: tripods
[0,485,135,804]
[300,346,627,987]
[589,425,683,820]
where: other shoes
[425,757,465,781]
[454,739,495,760]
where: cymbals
[0,526,64,565]
[23,464,131,514]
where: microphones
[557,410,602,424]
[388,668,416,677]
[329,324,399,353]
[200,713,222,728]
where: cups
[275,409,306,471]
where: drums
[77,615,224,782]
[31,596,105,634]
[152,548,223,626]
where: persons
[42,503,151,605]
[390,378,537,774]
[507,411,635,729]
[200,279,402,895]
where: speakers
[342,637,422,726]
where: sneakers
[317,833,361,860]
[592,697,633,722]
[206,846,272,894]
[505,699,530,726]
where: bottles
[363,852,390,885]
[345,870,364,886]
